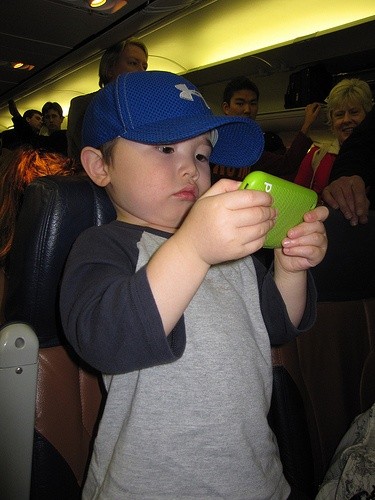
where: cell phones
[238,170,318,248]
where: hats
[83,70,265,168]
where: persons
[0,145,78,325]
[59,70,329,500]
[66,38,148,159]
[222,75,375,226]
[0,92,68,156]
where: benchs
[1,178,375,500]
